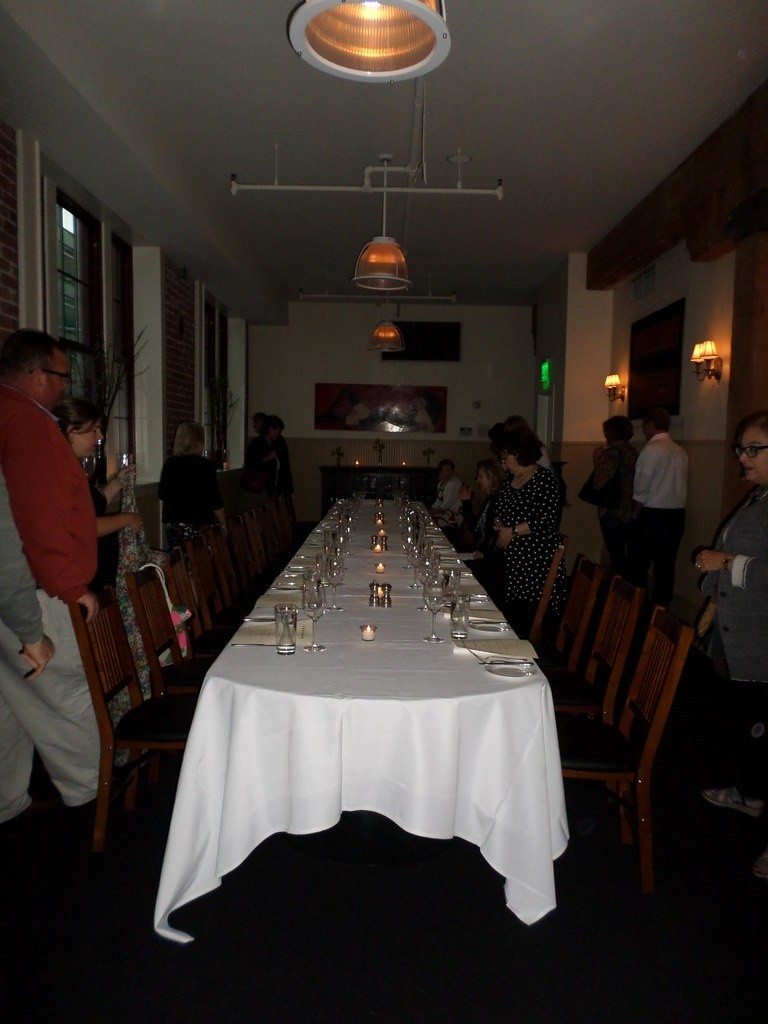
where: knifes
[478,662,534,666]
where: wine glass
[391,488,447,642]
[303,489,367,652]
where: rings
[697,566,701,568]
[695,563,697,566]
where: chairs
[68,491,294,852]
[524,534,693,894]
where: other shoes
[23,796,56,818]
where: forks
[468,649,528,662]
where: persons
[158,421,228,554]
[0,327,158,853]
[591,416,638,571]
[240,412,295,516]
[432,414,568,637]
[626,407,689,613]
[695,409,768,878]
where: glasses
[30,368,72,385]
[735,446,768,456]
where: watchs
[512,525,520,537]
[722,558,730,571]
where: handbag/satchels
[578,448,624,510]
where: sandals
[754,849,768,879]
[703,786,761,817]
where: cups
[452,594,470,639]
[274,604,298,654]
[117,452,133,469]
[444,569,460,608]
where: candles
[375,544,381,552]
[377,586,384,598]
[376,519,382,525]
[379,529,385,536]
[375,563,385,573]
[361,624,374,640]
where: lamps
[288,0,450,84]
[690,340,722,381]
[351,154,413,291]
[604,375,625,402]
[367,291,404,352]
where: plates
[416,506,538,677]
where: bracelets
[221,526,226,528]
